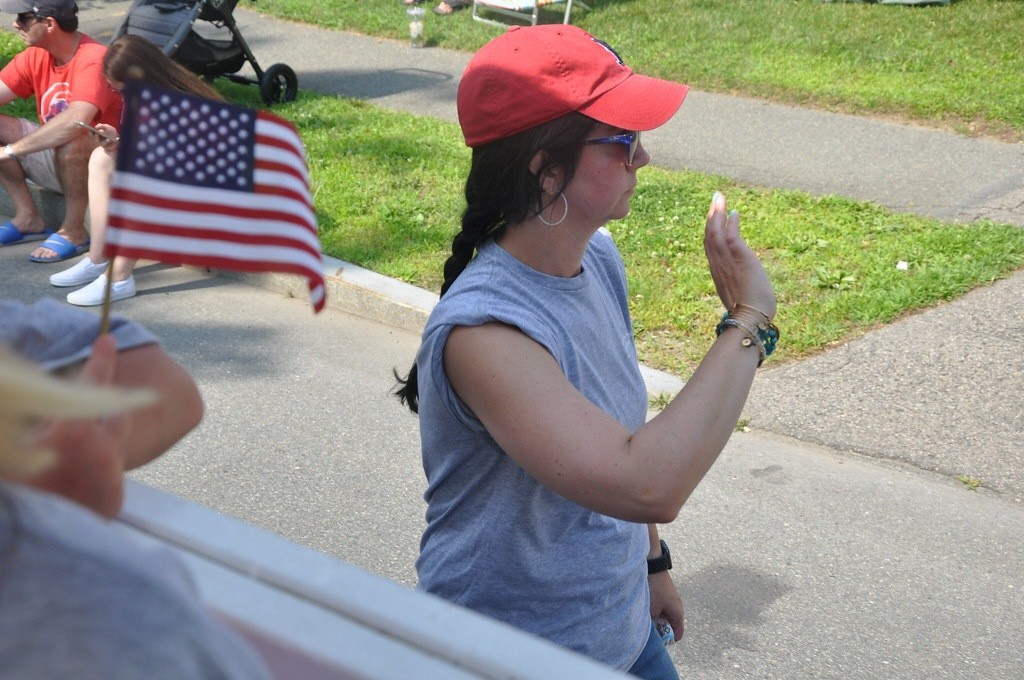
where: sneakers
[66,273,137,306]
[50,256,110,285]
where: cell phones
[73,121,117,143]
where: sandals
[404,0,430,5]
[434,0,471,15]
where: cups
[408,7,427,48]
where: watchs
[5,143,16,161]
[649,540,672,575]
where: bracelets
[716,303,778,368]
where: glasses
[18,14,35,23]
[580,130,639,163]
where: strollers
[106,0,301,112]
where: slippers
[28,233,90,263]
[1,220,51,245]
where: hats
[457,24,688,146]
[1,0,79,19]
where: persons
[414,23,779,679]
[0,0,124,263]
[401,0,470,13]
[49,34,233,307]
[0,333,279,679]
[2,291,205,476]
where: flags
[104,71,326,315]
